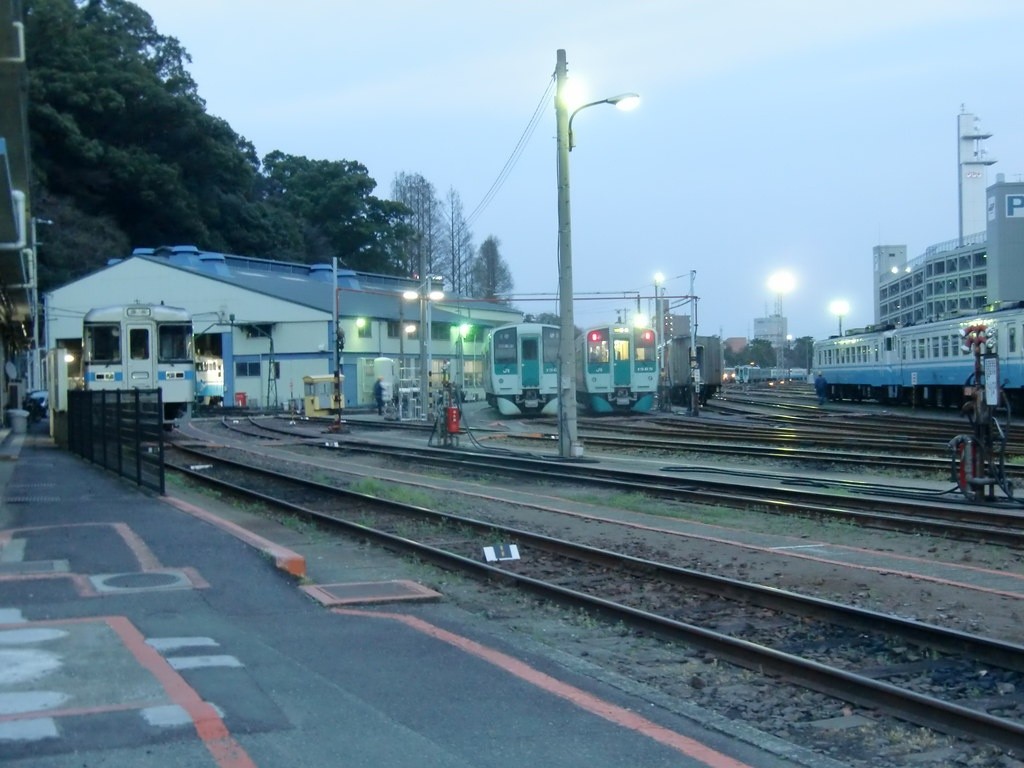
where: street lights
[831,299,848,337]
[54,346,75,412]
[402,275,445,421]
[398,308,416,379]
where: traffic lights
[357,316,373,338]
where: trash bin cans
[8,410,30,433]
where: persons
[371,377,388,416]
[814,371,830,406]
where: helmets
[378,376,384,381]
[817,371,822,376]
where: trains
[814,299,1024,413]
[81,300,227,430]
[735,364,808,383]
[575,310,659,413]
